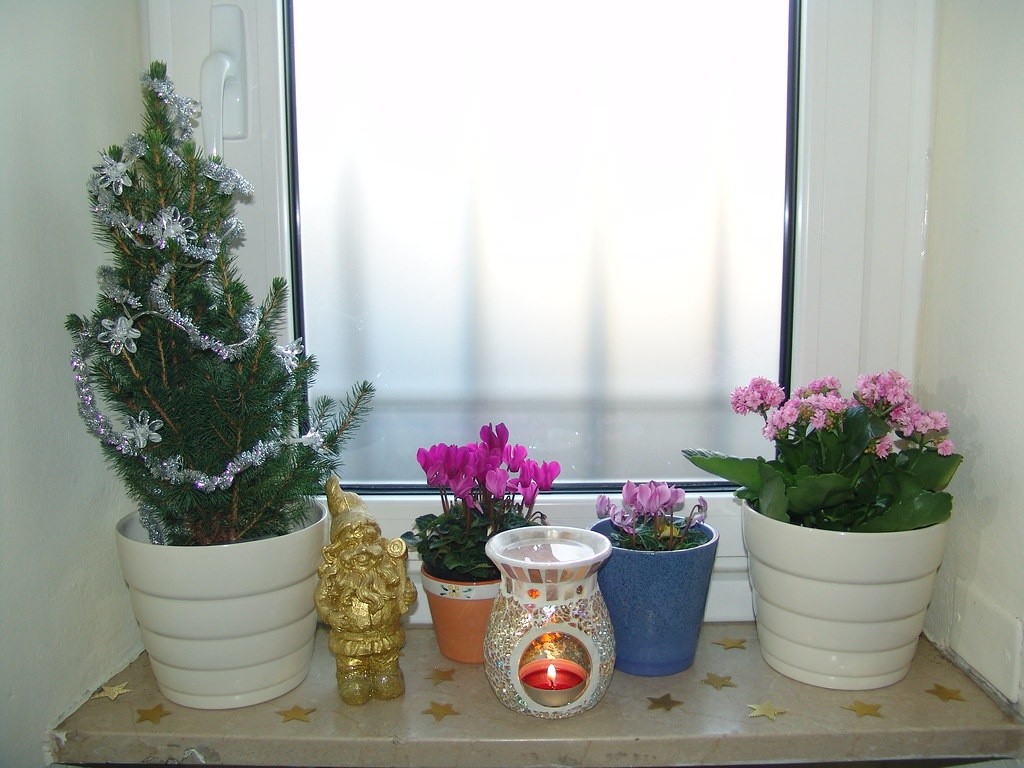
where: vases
[589,516,719,677]
[420,562,501,665]
[741,499,953,691]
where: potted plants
[61,58,376,711]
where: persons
[313,477,418,705]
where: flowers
[399,422,560,582]
[596,478,709,551]
[680,368,964,532]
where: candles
[519,659,587,707]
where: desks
[48,620,1024,768]
